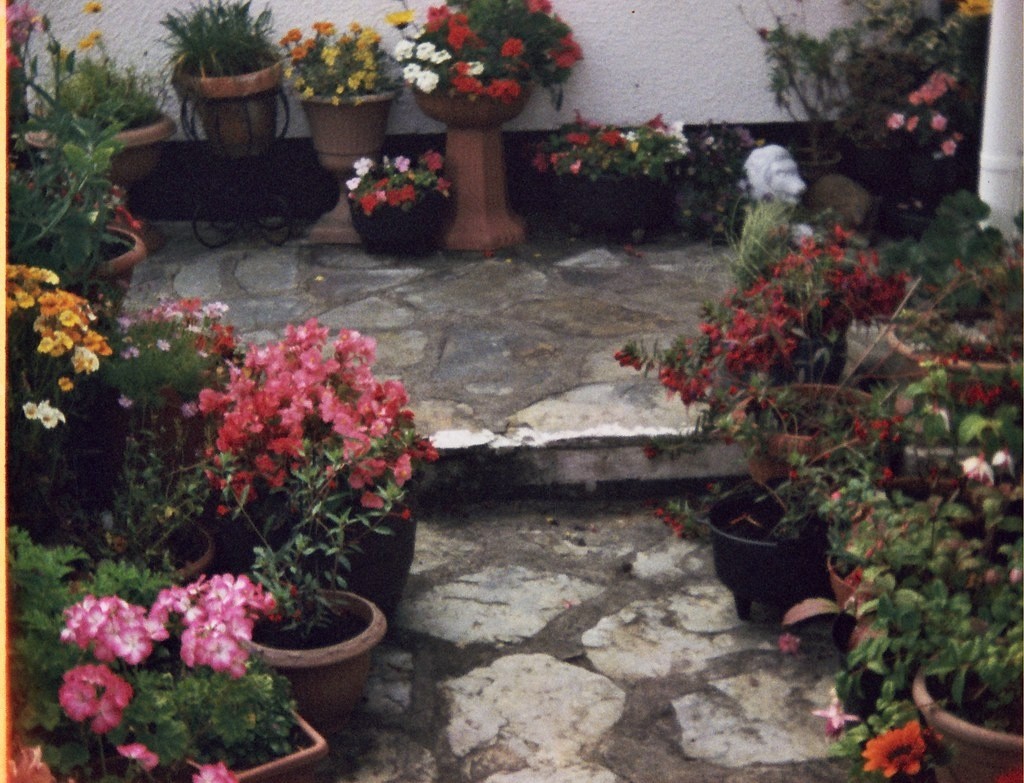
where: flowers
[346,148,450,218]
[6,0,169,135]
[615,189,1024,783]
[8,124,437,783]
[279,22,406,108]
[533,0,993,184]
[386,0,583,112]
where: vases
[414,84,528,129]
[550,172,741,233]
[181,707,329,783]
[796,145,839,180]
[173,512,216,584]
[709,489,831,630]
[764,334,849,384]
[887,190,940,234]
[294,89,396,171]
[826,554,873,616]
[733,383,874,486]
[913,647,1024,783]
[348,195,453,256]
[23,116,178,188]
[313,495,418,618]
[250,589,388,738]
[67,226,147,314]
[833,608,911,729]
[885,476,987,539]
[888,307,1023,385]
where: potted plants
[142,0,288,162]
